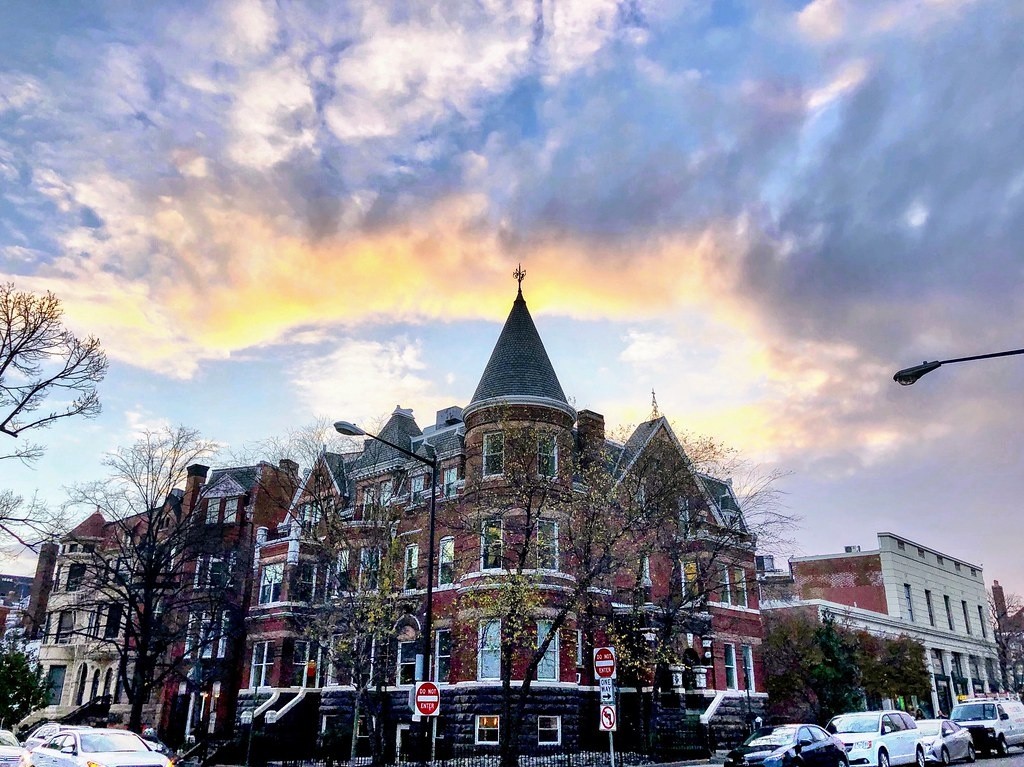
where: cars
[139,734,185,767]
[1,730,33,767]
[29,728,175,767]
[25,724,93,752]
[913,718,976,767]
[826,710,925,766]
[724,723,849,767]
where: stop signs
[593,647,617,680]
[414,682,440,716]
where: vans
[949,693,1024,758]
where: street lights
[333,419,438,766]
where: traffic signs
[600,678,614,703]
